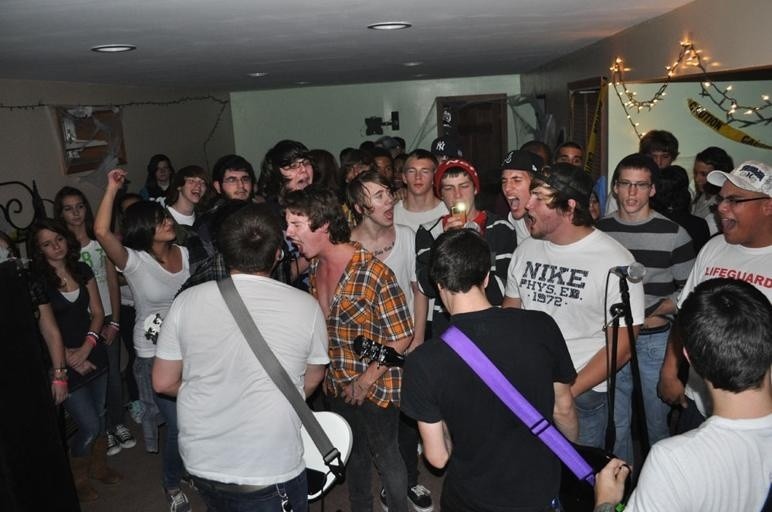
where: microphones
[608,262,646,283]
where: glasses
[185,179,206,187]
[715,196,768,206]
[618,181,651,191]
[225,177,251,184]
[285,158,311,170]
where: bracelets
[594,502,616,512]
[52,369,68,374]
[86,335,97,345]
[51,379,68,385]
[109,320,121,327]
[107,324,120,332]
[52,375,68,380]
[293,255,301,278]
[87,330,100,341]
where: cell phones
[450,205,466,226]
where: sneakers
[105,429,123,456]
[107,422,137,449]
[379,488,389,510]
[127,400,146,424]
[162,481,192,512]
[406,483,434,512]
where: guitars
[351,335,633,511]
[143,313,355,503]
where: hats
[706,161,772,199]
[431,136,456,155]
[432,157,481,199]
[501,150,543,172]
[535,163,593,205]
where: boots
[69,447,94,486]
[91,443,122,484]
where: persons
[655,158,772,437]
[0,231,83,510]
[309,149,343,203]
[151,205,333,512]
[497,150,546,248]
[194,155,268,231]
[591,278,771,512]
[393,148,453,341]
[163,164,210,243]
[175,200,294,301]
[115,193,143,425]
[80,111,118,167]
[392,153,410,191]
[430,135,464,165]
[279,183,414,512]
[586,187,599,223]
[339,137,407,202]
[592,153,700,481]
[601,128,710,218]
[257,139,314,206]
[94,167,197,511]
[549,140,584,169]
[399,226,580,512]
[24,216,123,503]
[649,166,710,245]
[344,170,418,512]
[140,154,178,206]
[499,166,644,451]
[686,146,733,240]
[53,186,138,457]
[401,157,518,356]
[515,141,553,174]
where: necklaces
[58,271,67,281]
